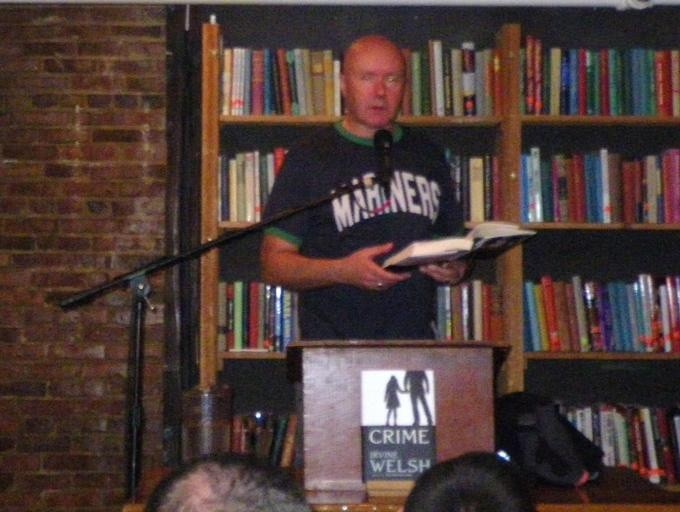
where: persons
[405,452,532,511]
[149,452,307,510]
[261,40,471,337]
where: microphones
[372,127,397,198]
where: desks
[126,466,677,512]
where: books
[224,39,502,116]
[549,393,679,482]
[217,277,504,352]
[520,37,678,117]
[523,273,679,353]
[383,221,537,270]
[211,378,296,468]
[521,147,678,222]
[223,146,500,228]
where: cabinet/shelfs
[179,6,680,397]
